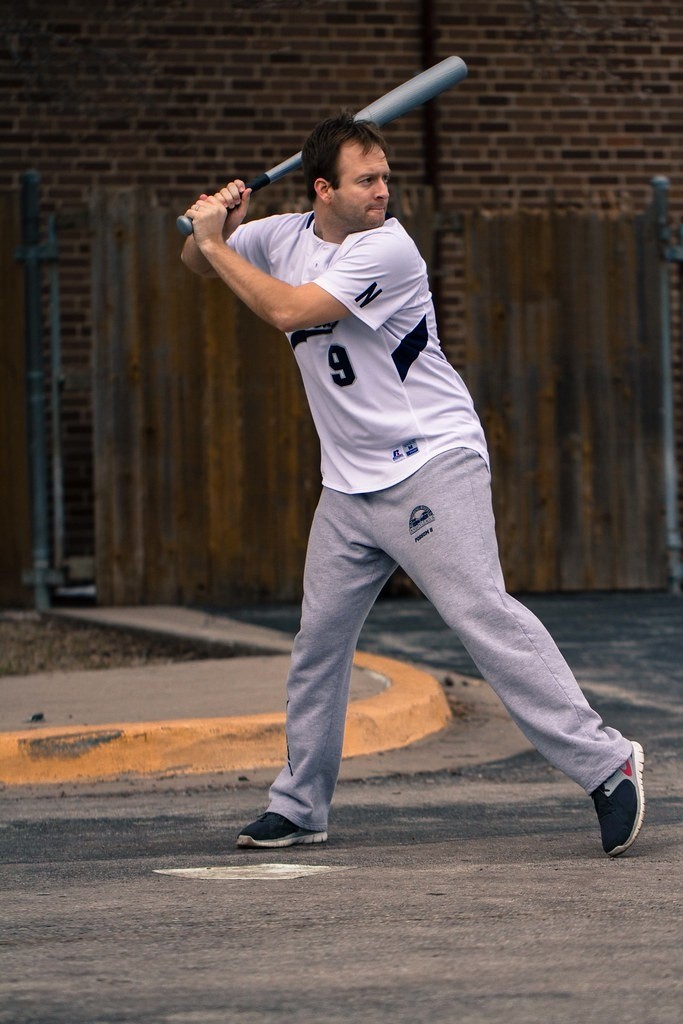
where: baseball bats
[176,54,469,237]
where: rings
[195,205,200,211]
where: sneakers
[592,741,645,854]
[235,811,328,849]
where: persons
[180,118,645,856]
